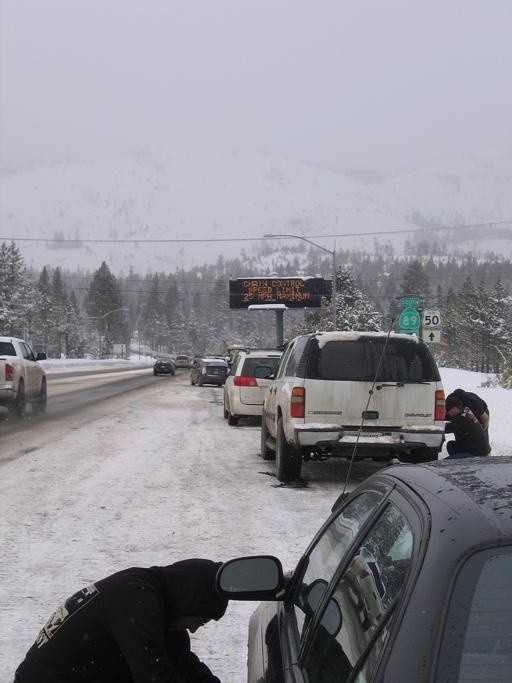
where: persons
[444,388,492,459]
[13,558,229,683]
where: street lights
[264,234,337,331]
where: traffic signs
[402,306,441,343]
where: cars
[0,336,46,416]
